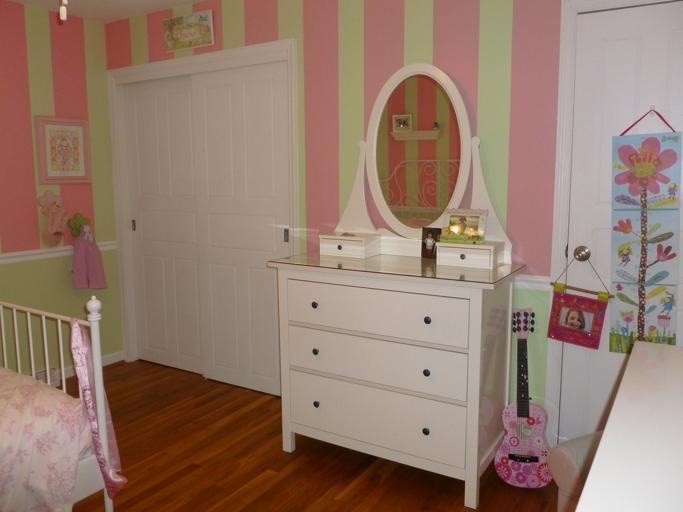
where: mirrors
[318,62,513,269]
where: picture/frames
[161,9,215,54]
[437,207,488,244]
[35,116,93,185]
[392,113,413,130]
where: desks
[574,339,683,512]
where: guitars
[493,307,555,489]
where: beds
[1,295,113,512]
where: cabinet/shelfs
[267,246,527,508]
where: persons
[423,231,435,259]
[561,309,585,330]
